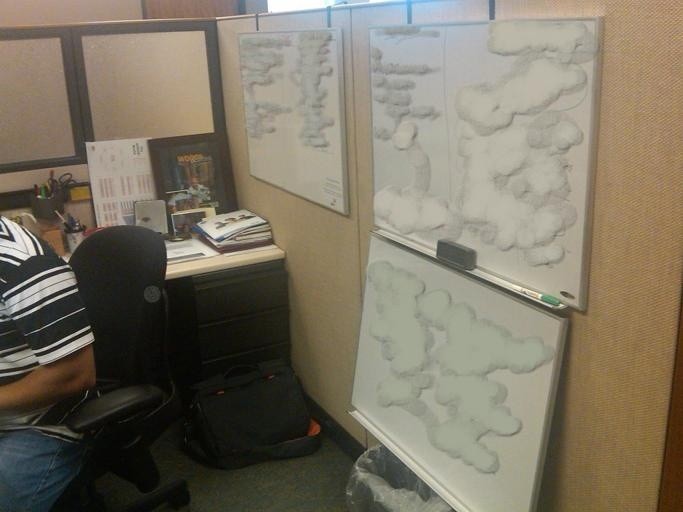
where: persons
[0,215,110,512]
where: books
[192,209,273,254]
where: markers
[520,287,561,308]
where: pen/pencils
[34,179,58,199]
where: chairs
[45,223,191,512]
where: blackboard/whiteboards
[351,232,570,511]
[237,28,351,217]
[367,19,602,316]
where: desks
[57,230,289,390]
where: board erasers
[436,239,476,270]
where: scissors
[58,172,76,191]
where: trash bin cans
[353,445,452,511]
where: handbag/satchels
[178,357,312,451]
